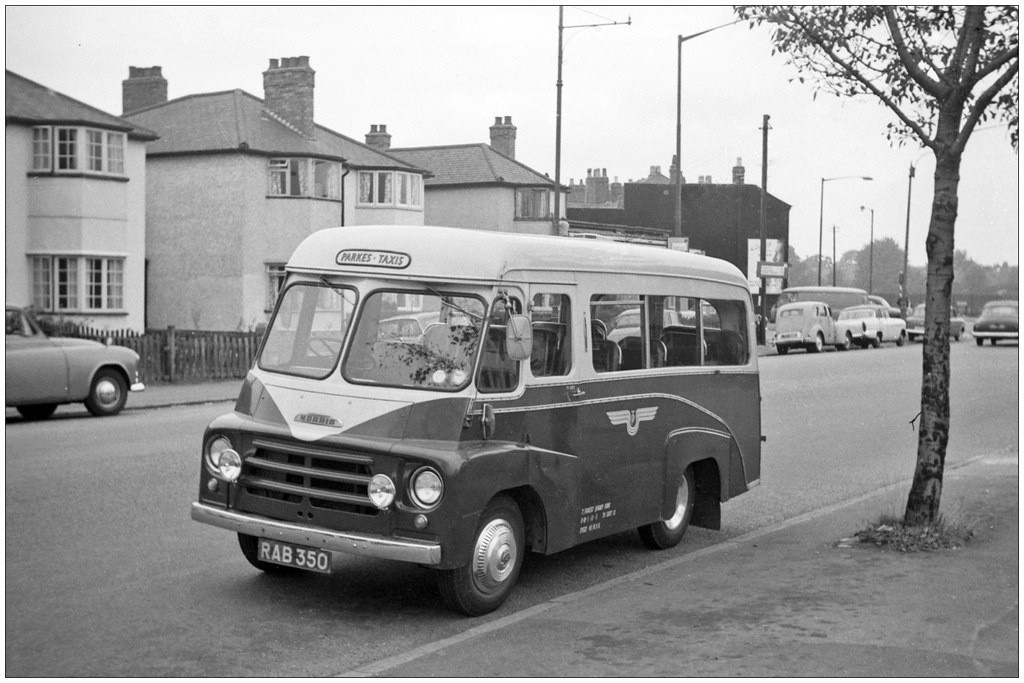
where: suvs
[773,301,852,353]
[837,304,908,348]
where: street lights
[818,176,873,283]
[671,10,788,240]
[860,205,874,297]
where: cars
[972,300,1019,346]
[906,303,966,342]
[5,306,146,416]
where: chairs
[375,316,747,383]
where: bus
[187,224,767,616]
[770,286,867,322]
